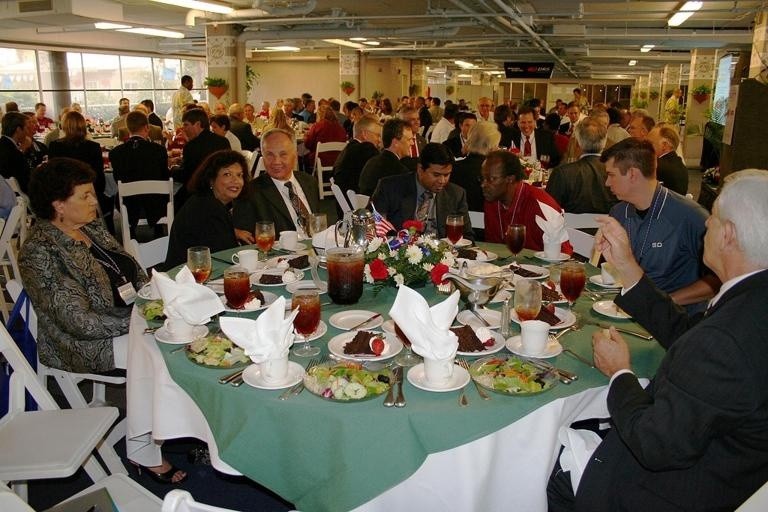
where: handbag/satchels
[0,288,39,412]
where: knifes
[593,320,654,341]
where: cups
[541,173,549,185]
[102,151,109,168]
[541,155,551,171]
[172,148,180,158]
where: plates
[135,209,639,408]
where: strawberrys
[485,339,495,346]
[547,280,556,290]
[543,303,555,313]
[372,333,387,356]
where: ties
[411,137,417,158]
[283,180,312,233]
[524,134,532,157]
[413,190,434,228]
[569,125,575,135]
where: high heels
[127,458,189,486]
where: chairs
[0,136,767,510]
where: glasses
[476,175,504,184]
